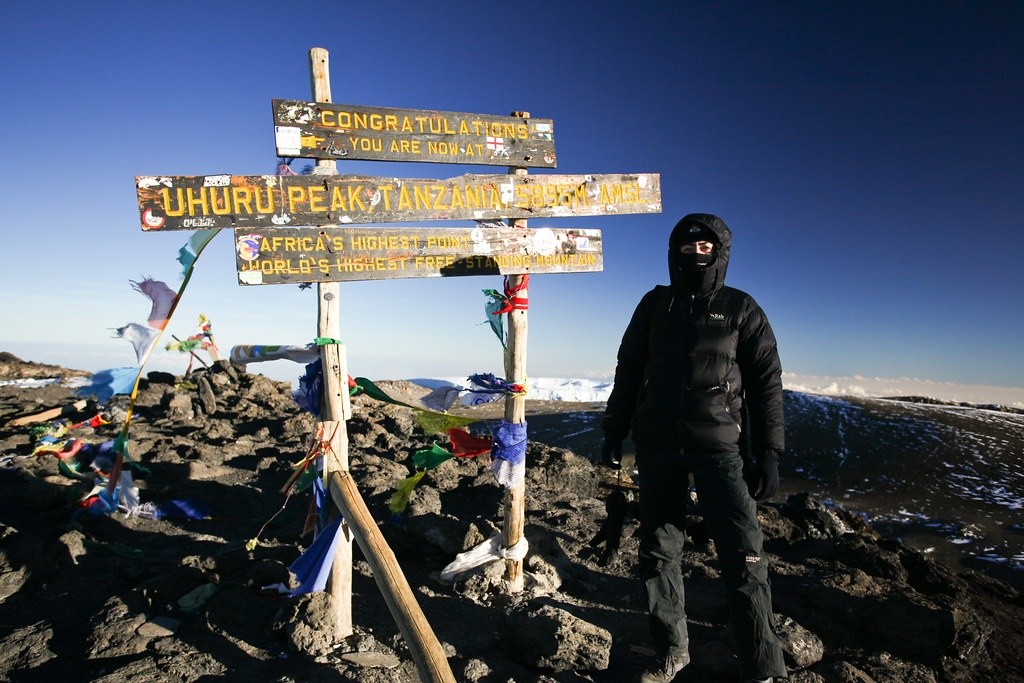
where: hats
[672,221,722,248]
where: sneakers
[642,649,690,683]
[748,676,773,683]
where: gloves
[745,448,780,502]
[601,433,623,470]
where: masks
[683,253,715,268]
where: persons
[601,212,786,683]
